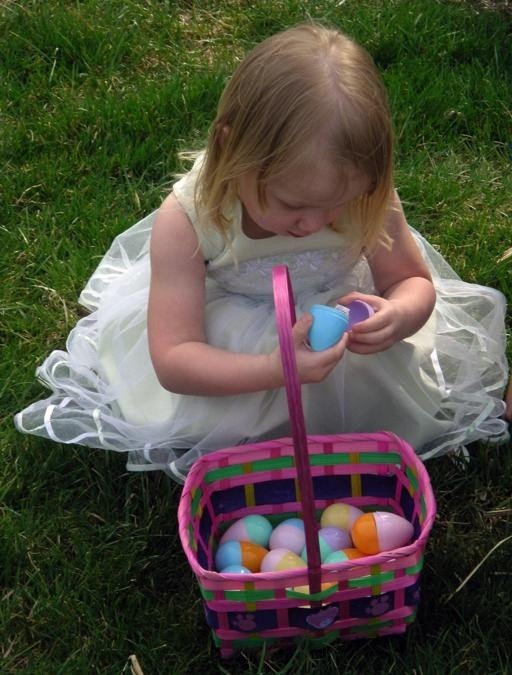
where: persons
[15,20,509,488]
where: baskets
[177,265,436,660]
